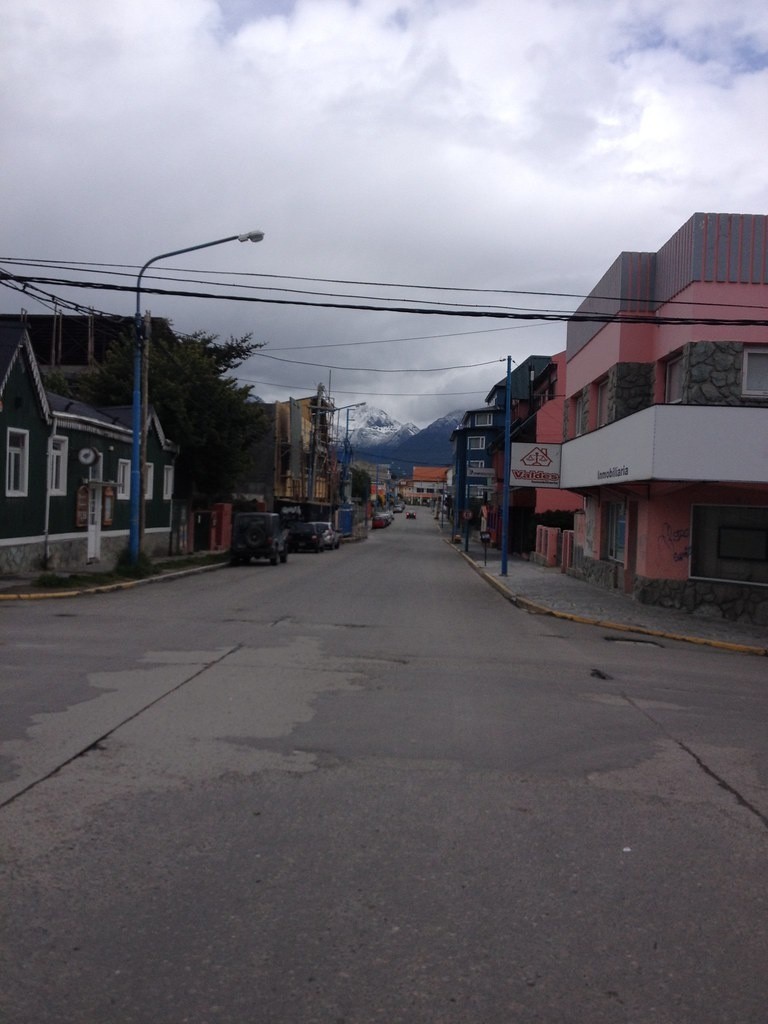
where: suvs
[230,512,287,567]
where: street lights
[127,230,268,564]
[306,402,367,496]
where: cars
[372,497,406,531]
[406,510,417,519]
[305,521,343,551]
[287,524,325,555]
